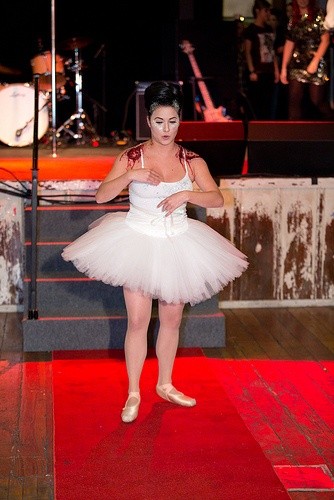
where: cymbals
[61,37,93,50]
[0,66,21,75]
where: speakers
[174,120,334,180]
[136,92,151,140]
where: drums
[30,51,66,91]
[0,83,50,147]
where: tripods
[45,48,102,149]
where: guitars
[179,40,233,122]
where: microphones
[16,129,21,136]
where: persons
[241,0,334,121]
[60,81,250,423]
